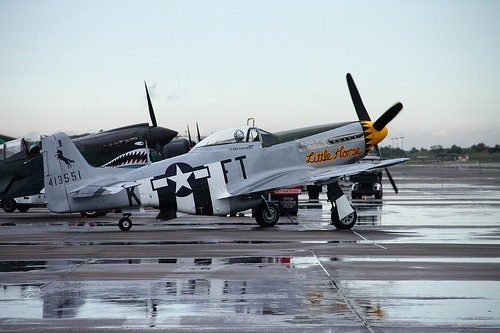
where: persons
[28,140,43,155]
[234,129,245,143]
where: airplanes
[40,72,403,233]
[0,79,177,213]
